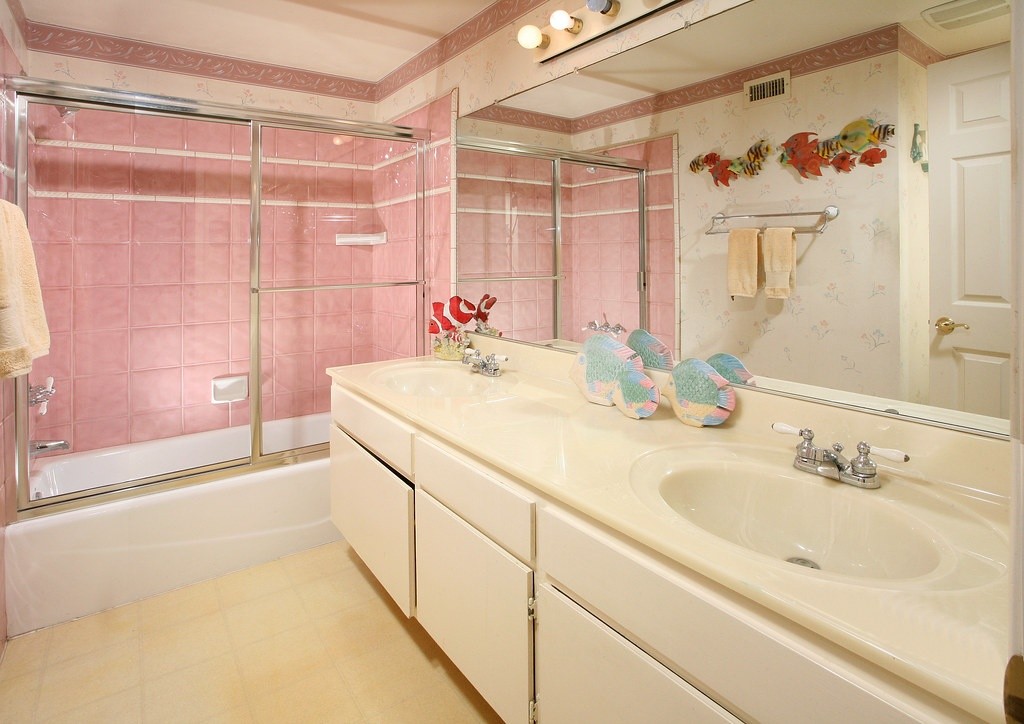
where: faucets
[772,423,910,489]
[462,347,509,377]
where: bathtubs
[6,412,330,630]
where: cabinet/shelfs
[536,491,984,724]
[416,429,538,724]
[331,384,415,619]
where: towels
[726,226,763,300]
[0,199,52,380]
[762,227,798,301]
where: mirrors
[456,0,1012,441]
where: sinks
[630,439,942,581]
[366,358,518,409]
